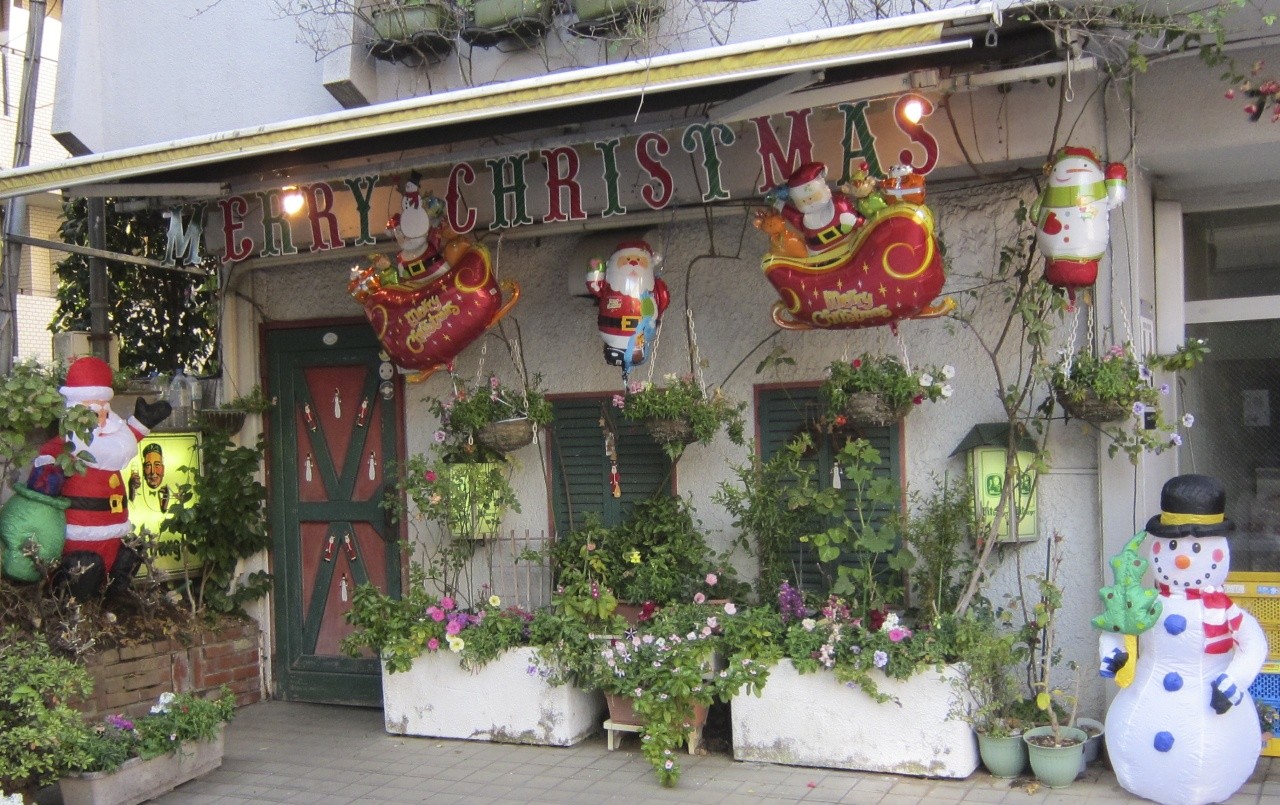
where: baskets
[473,414,532,452]
[1055,385,1140,423]
[646,414,699,445]
[842,392,905,426]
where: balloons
[1028,147,1127,285]
[751,162,956,327]
[589,240,672,366]
[347,218,506,386]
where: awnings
[1,0,1108,277]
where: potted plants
[567,495,749,788]
[425,370,555,460]
[930,529,1105,794]
[1042,334,1221,461]
[192,381,275,439]
[615,368,755,458]
[43,680,239,803]
[813,350,962,428]
